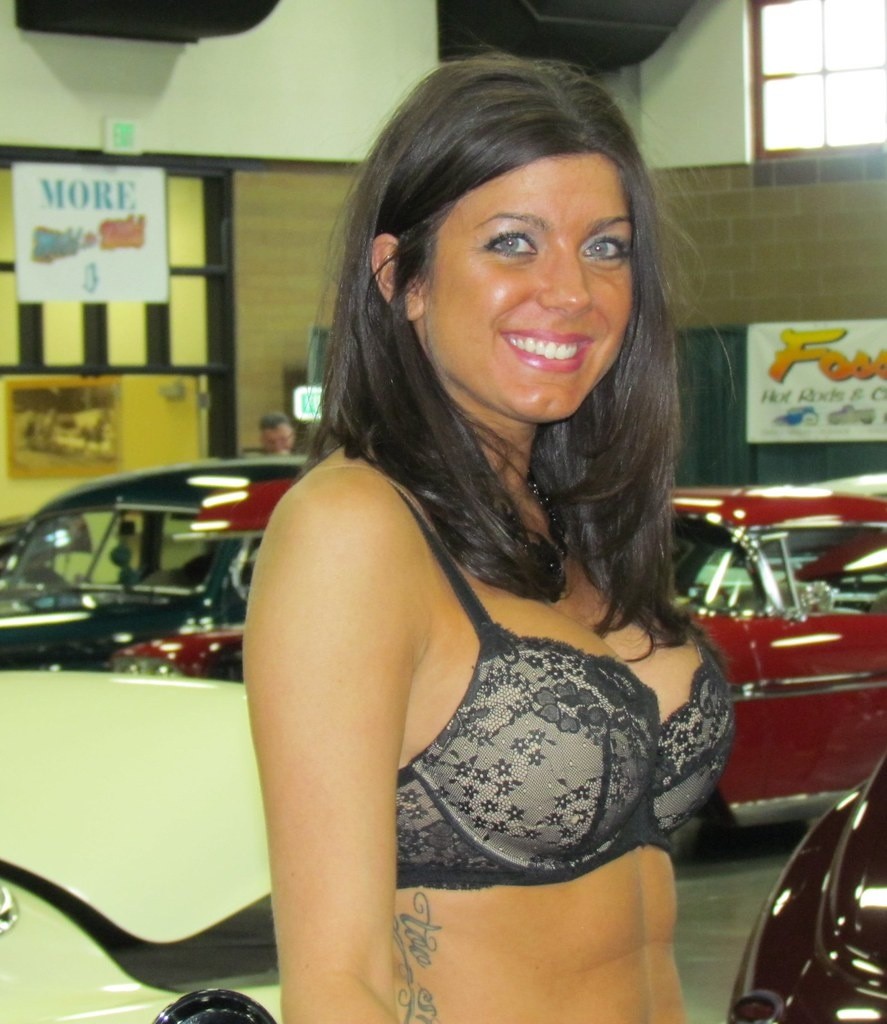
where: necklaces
[503,467,568,570]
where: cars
[1,663,285,1024]
[108,476,887,867]
[0,454,310,674]
[729,755,887,1024]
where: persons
[257,414,293,455]
[243,57,735,1024]
